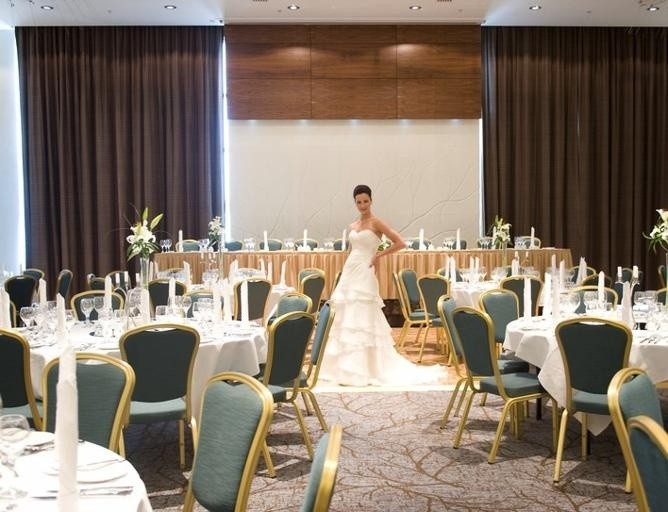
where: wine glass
[19,295,220,347]
[159,236,527,253]
[201,269,255,289]
[551,289,668,338]
[459,266,576,290]
[0,414,32,479]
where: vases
[500,241,508,267]
[139,257,149,286]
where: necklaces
[354,216,376,225]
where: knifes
[30,483,134,499]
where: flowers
[124,205,163,261]
[209,216,225,251]
[492,215,513,246]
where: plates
[75,456,129,484]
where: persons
[317,184,407,386]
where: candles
[456,228,460,250]
[342,229,347,251]
[263,230,269,251]
[179,230,183,253]
[418,229,424,250]
[530,227,535,249]
[304,229,308,252]
